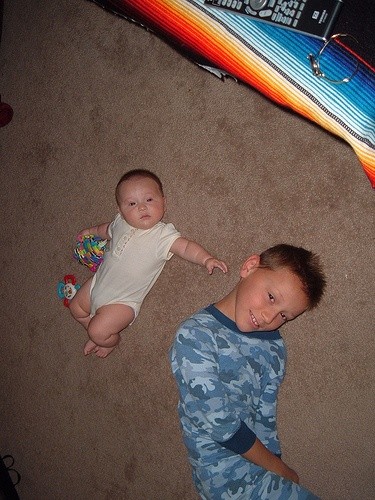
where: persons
[168,243,328,500]
[67,169,229,360]
